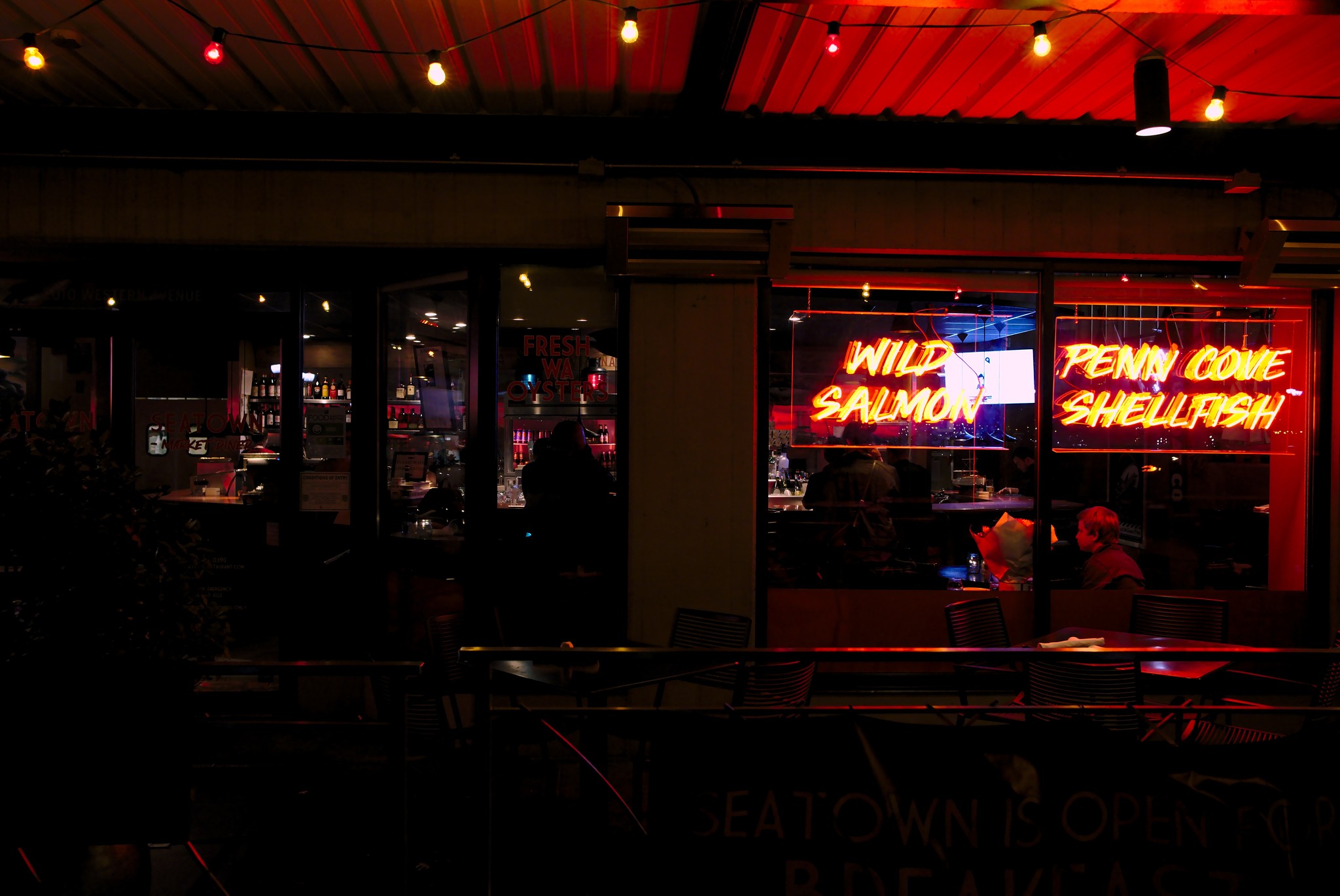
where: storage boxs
[205,488,220,495]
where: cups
[797,411,811,429]
[497,486,526,506]
[986,480,994,496]
[833,426,844,437]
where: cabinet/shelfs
[503,414,617,476]
[249,399,466,435]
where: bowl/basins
[817,437,828,444]
[797,433,817,444]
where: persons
[1076,506,1146,591]
[803,436,931,520]
[522,420,616,575]
[237,427,278,477]
[995,446,1035,498]
[815,424,900,566]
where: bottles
[454,406,466,431]
[250,371,280,399]
[396,374,421,400]
[768,442,808,496]
[598,424,609,444]
[303,403,353,428]
[600,451,615,471]
[967,553,989,575]
[389,407,424,429]
[251,403,280,428]
[303,372,351,400]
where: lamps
[1134,52,1173,135]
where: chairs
[944,593,1340,747]
[653,608,817,722]
[404,612,479,752]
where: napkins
[1037,637,1105,649]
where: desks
[939,564,1073,591]
[494,639,739,707]
[390,524,464,610]
[1010,627,1252,744]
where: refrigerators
[503,394,617,493]
